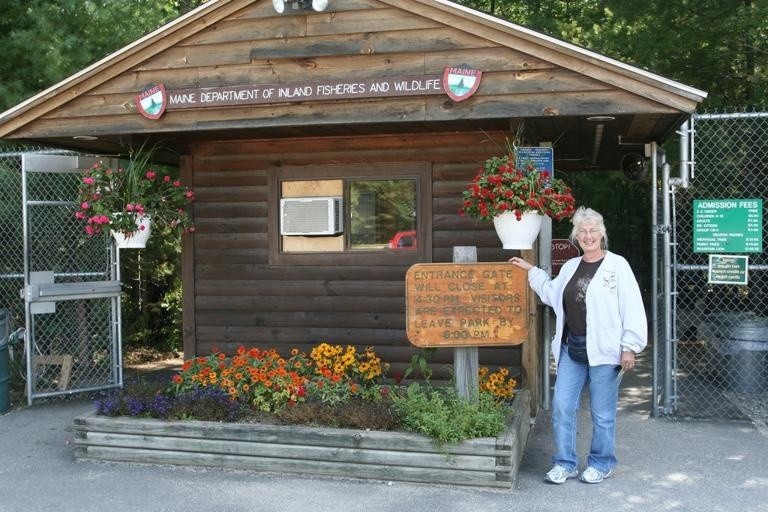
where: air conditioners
[278,194,344,236]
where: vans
[388,230,417,251]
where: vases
[106,209,156,250]
[490,203,545,251]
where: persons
[509,205,648,485]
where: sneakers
[544,462,579,484]
[580,466,613,484]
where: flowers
[70,158,198,252]
[458,147,575,226]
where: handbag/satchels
[568,343,588,363]
